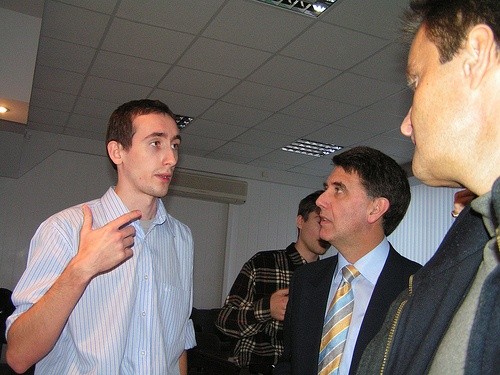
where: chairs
[190,307,231,349]
[0,288,14,362]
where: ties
[318,264,361,375]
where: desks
[186,332,240,374]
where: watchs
[451,210,459,218]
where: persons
[358,0,500,375]
[4,99,197,375]
[215,190,333,375]
[276,147,425,375]
[452,190,479,218]
